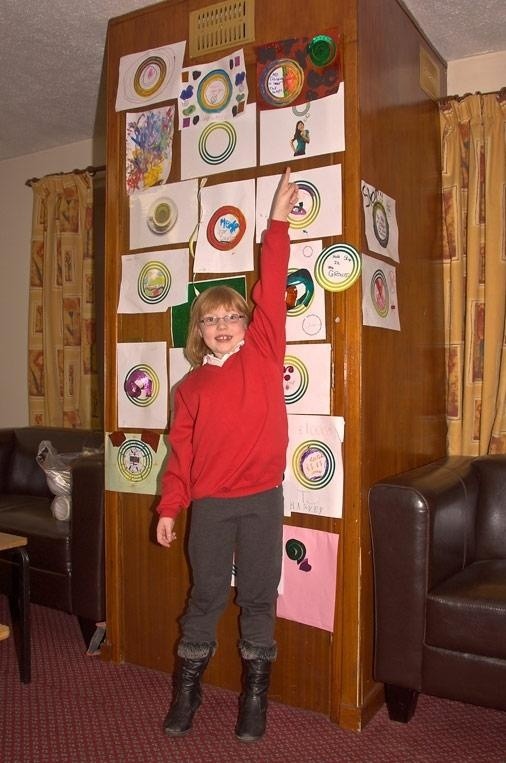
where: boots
[163,638,219,737]
[235,638,278,741]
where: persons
[290,121,311,155]
[155,165,301,742]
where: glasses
[198,313,246,325]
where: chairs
[0,427,104,650]
[369,454,506,722]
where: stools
[0,531,31,683]
